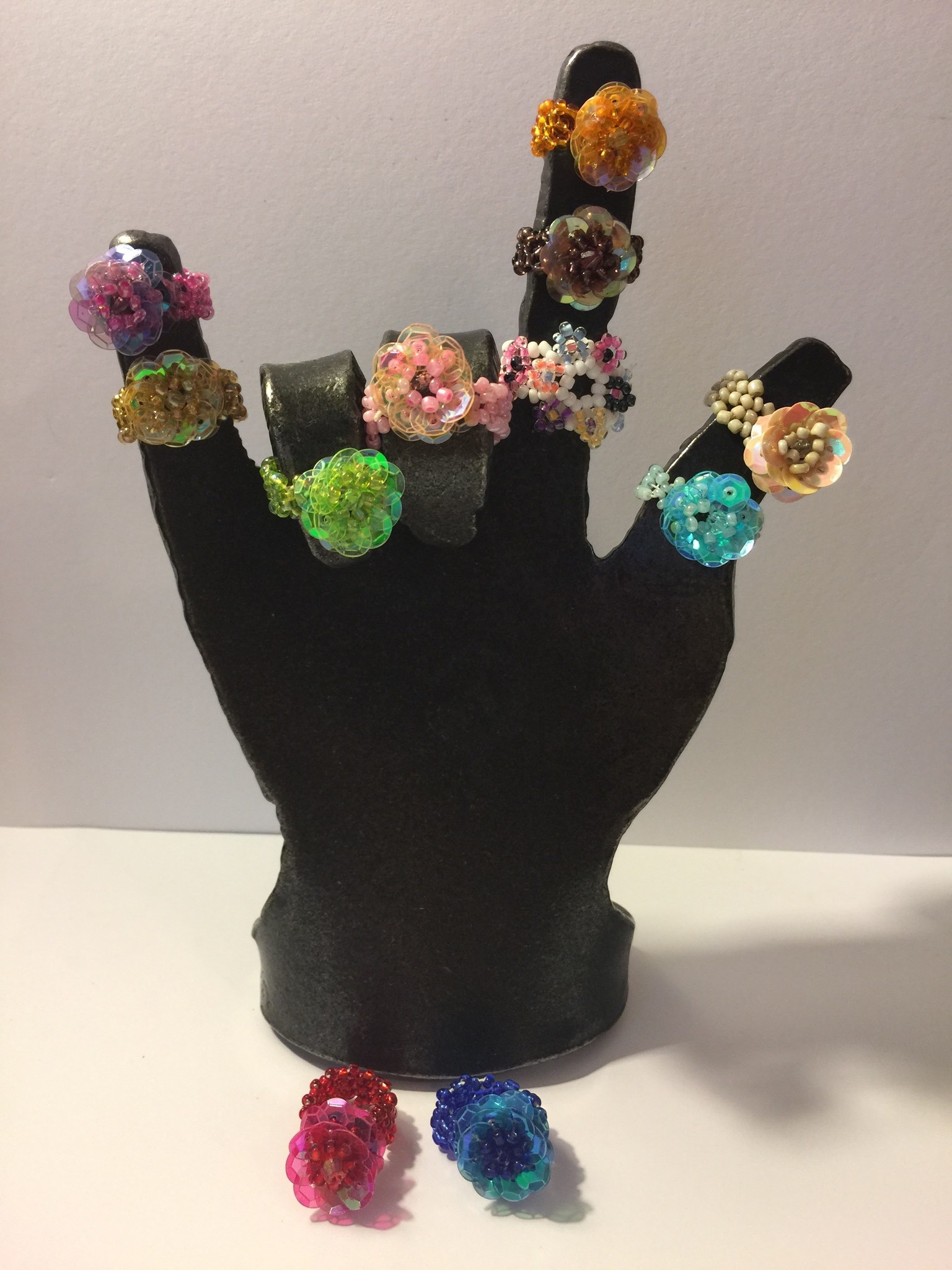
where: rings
[286,1064,400,1216]
[499,322,637,449]
[705,370,853,503]
[68,242,214,356]
[361,323,513,450]
[261,448,405,558]
[512,204,643,312]
[431,1074,554,1202]
[110,349,247,447]
[637,463,766,568]
[530,82,668,192]
